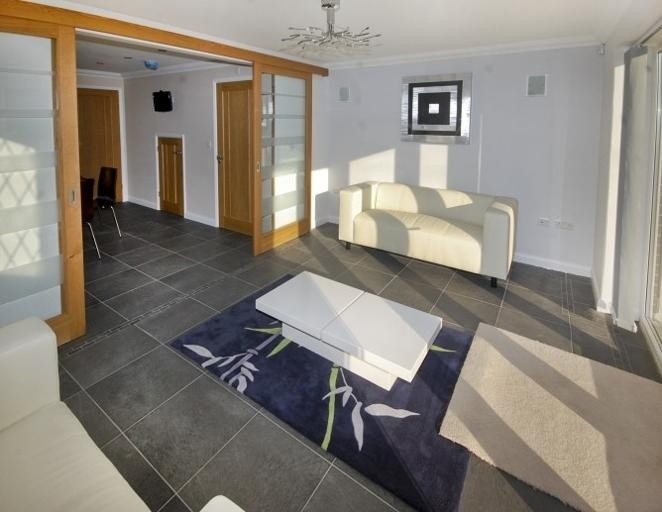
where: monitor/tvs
[152,90,172,112]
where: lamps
[280,1,382,57]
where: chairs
[80,167,123,262]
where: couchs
[338,179,518,288]
[0,316,244,512]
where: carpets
[438,319,662,512]
[164,274,471,512]
